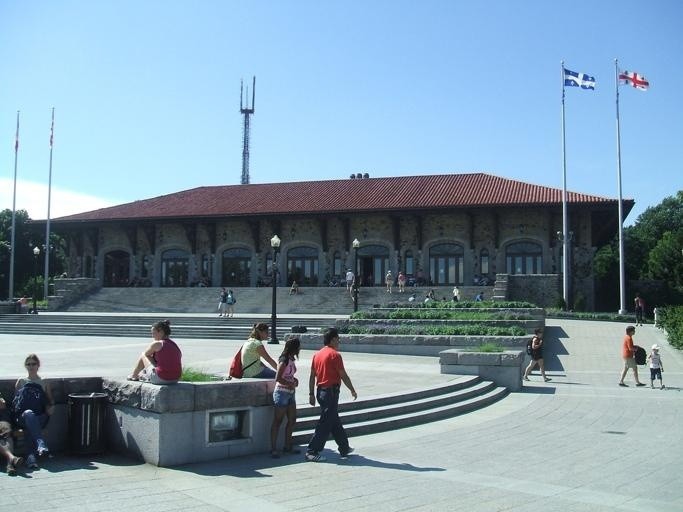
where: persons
[325,270,361,301]
[523,327,552,382]
[385,272,405,292]
[19,295,29,314]
[218,287,234,317]
[12,354,55,469]
[416,268,425,286]
[0,392,24,476]
[271,338,301,457]
[634,292,644,326]
[128,270,147,287]
[240,323,277,378]
[255,269,281,287]
[290,280,299,295]
[442,286,461,302]
[646,343,665,390]
[191,269,209,287]
[128,320,182,384]
[408,290,435,302]
[306,330,358,459]
[474,274,482,301]
[618,325,645,387]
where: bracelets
[309,393,314,395]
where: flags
[618,70,649,92]
[563,68,596,91]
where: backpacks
[13,382,51,417]
[526,335,540,356]
[228,344,259,378]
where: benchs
[0,413,49,456]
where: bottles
[528,345,531,353]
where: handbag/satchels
[0,421,14,439]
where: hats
[652,343,660,350]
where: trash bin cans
[67,393,108,456]
[15,303,20,313]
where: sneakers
[26,454,39,469]
[635,383,646,386]
[340,447,357,456]
[37,440,50,458]
[305,452,328,462]
[125,375,139,381]
[11,455,25,468]
[544,378,552,382]
[618,383,630,388]
[649,383,665,390]
[5,463,17,477]
[523,376,529,381]
[283,448,302,456]
[269,450,282,460]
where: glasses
[26,363,38,366]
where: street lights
[350,238,359,313]
[555,229,574,312]
[265,232,285,348]
[31,247,41,314]
